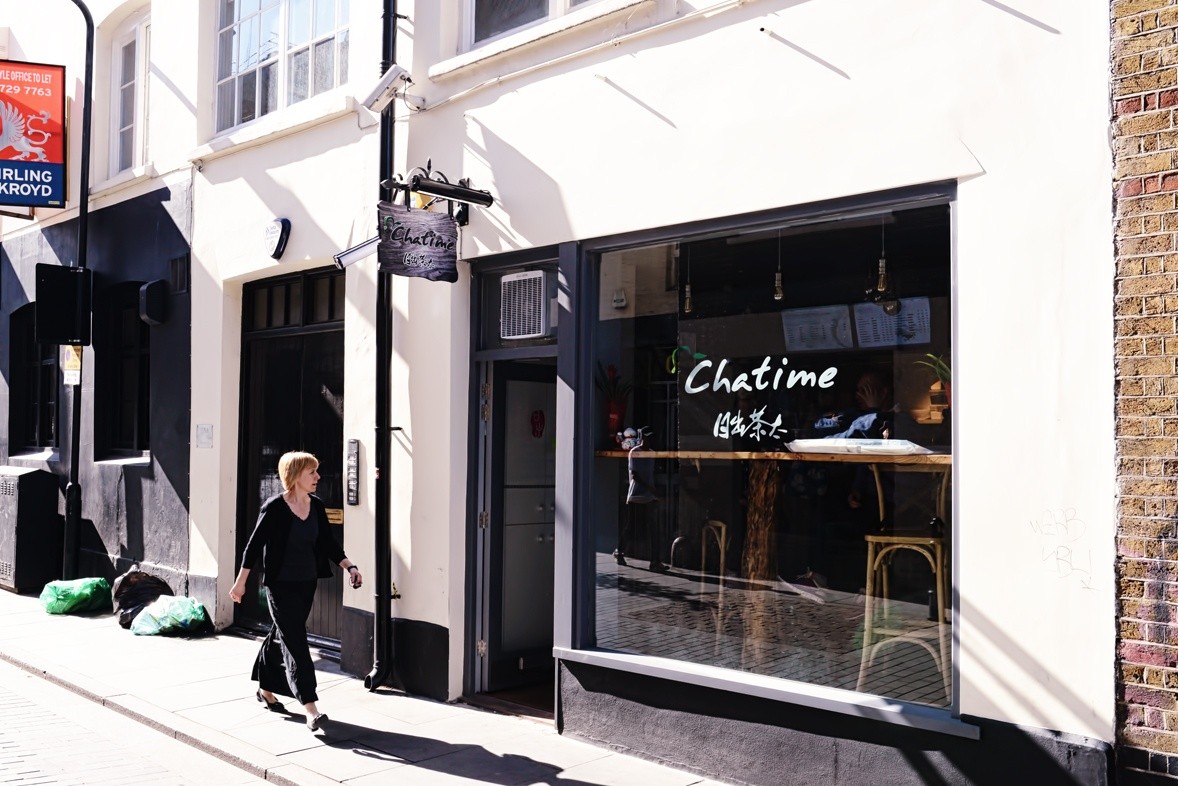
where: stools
[854,462,952,704]
[691,460,787,656]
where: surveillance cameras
[360,64,410,113]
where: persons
[228,451,362,732]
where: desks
[596,450,952,674]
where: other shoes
[257,691,285,712]
[308,714,328,732]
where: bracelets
[347,565,358,573]
